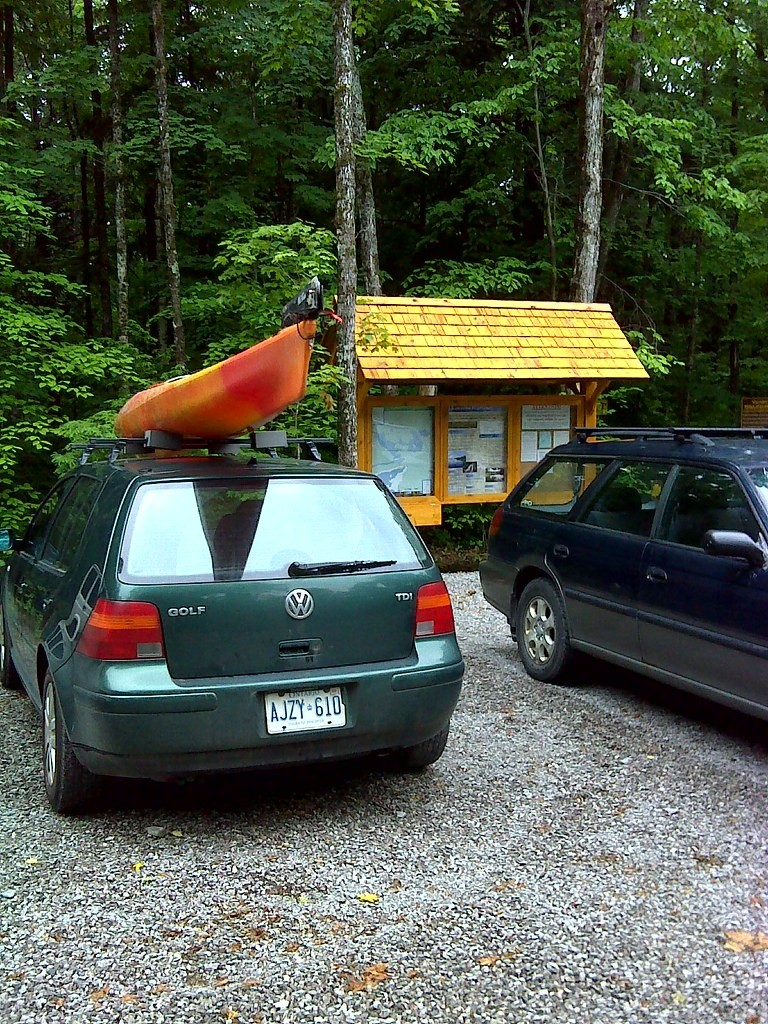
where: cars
[477,427,767,721]
[0,451,465,817]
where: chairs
[674,489,754,552]
[588,485,642,532]
[212,498,263,572]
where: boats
[114,271,324,451]
